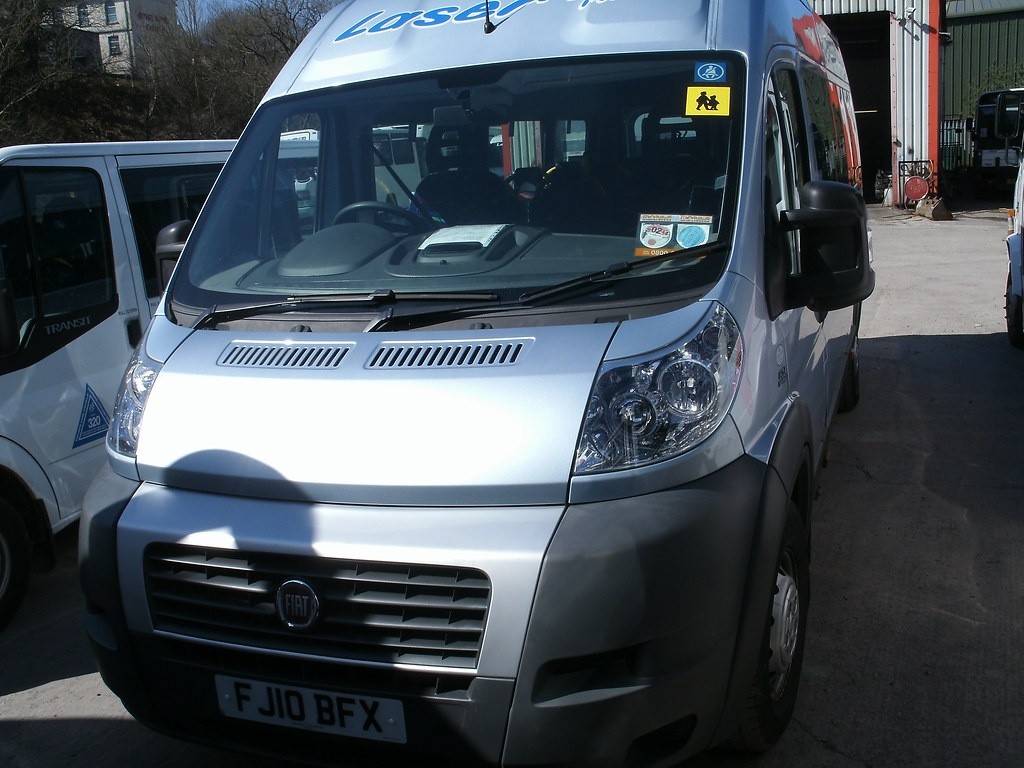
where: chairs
[641,107,708,162]
[408,120,528,227]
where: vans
[1003,130,1024,351]
[963,86,1024,200]
[73,2,879,768]
[0,128,377,641]
